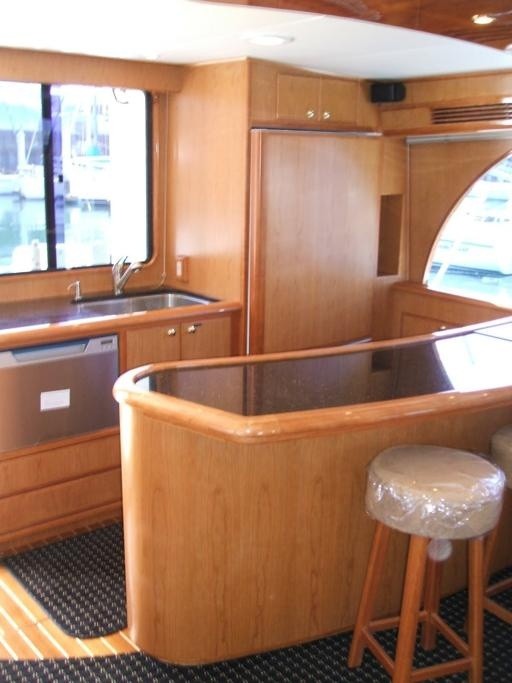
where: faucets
[112,253,144,296]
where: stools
[347,444,506,683]
[463,423,512,633]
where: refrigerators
[246,126,384,355]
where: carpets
[4,522,128,639]
[0,564,512,683]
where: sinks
[76,289,219,323]
